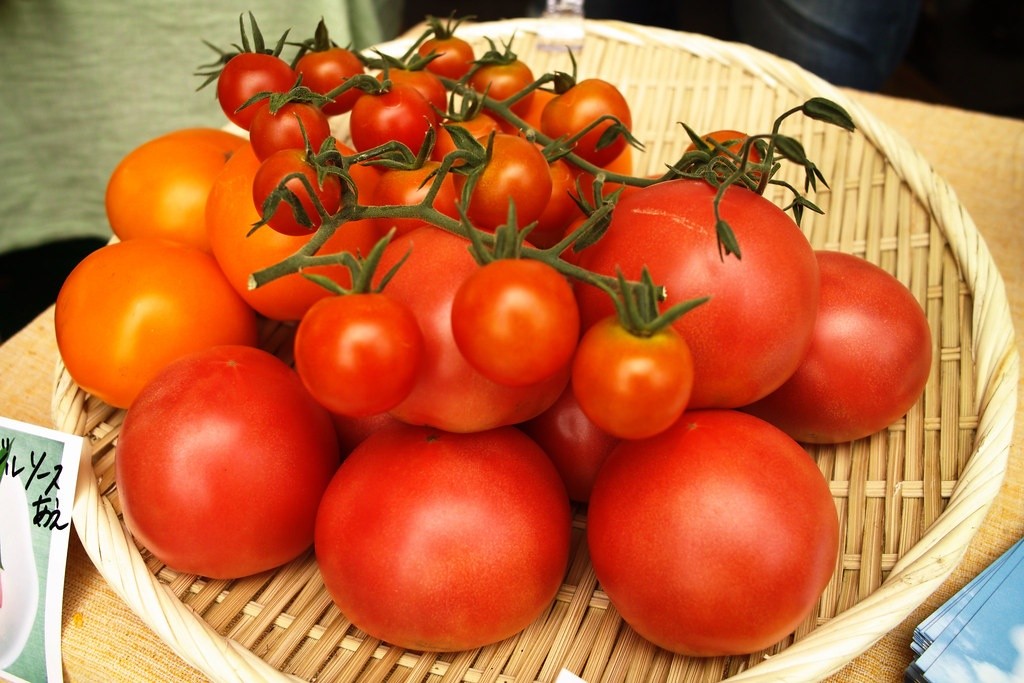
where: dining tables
[0,15,1023,682]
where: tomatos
[53,7,931,659]
